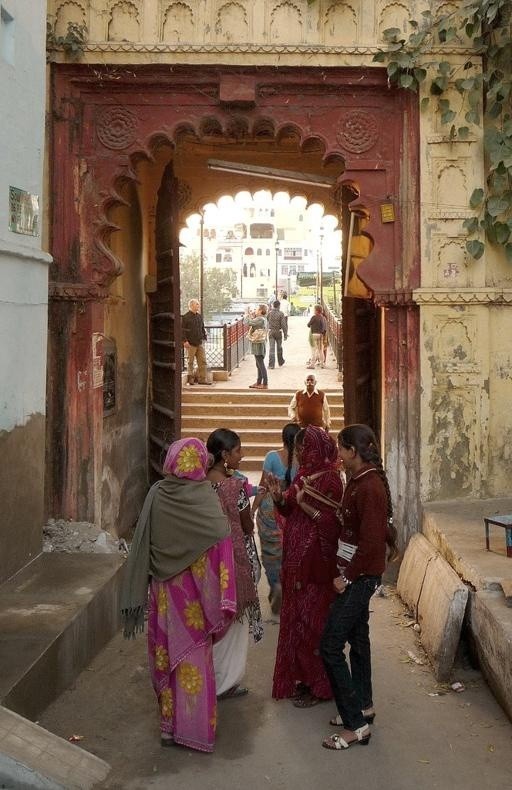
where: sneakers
[189,376,195,385]
[249,383,261,387]
[198,382,212,385]
[161,732,176,747]
[257,384,268,389]
[306,365,315,369]
[268,366,274,369]
[321,364,327,368]
[279,360,285,366]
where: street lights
[199,207,206,320]
[319,226,324,305]
[274,239,280,300]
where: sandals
[288,683,308,697]
[294,694,331,707]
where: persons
[244,288,293,392]
[182,300,212,385]
[205,428,264,701]
[225,467,266,496]
[306,313,330,365]
[121,438,237,754]
[288,374,331,433]
[265,425,343,708]
[251,424,302,613]
[318,424,400,749]
[306,305,325,369]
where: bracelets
[311,510,320,520]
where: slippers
[217,685,249,699]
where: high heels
[322,724,371,750]
[329,706,375,726]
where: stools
[485,514,512,556]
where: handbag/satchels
[246,329,267,343]
[336,486,345,526]
